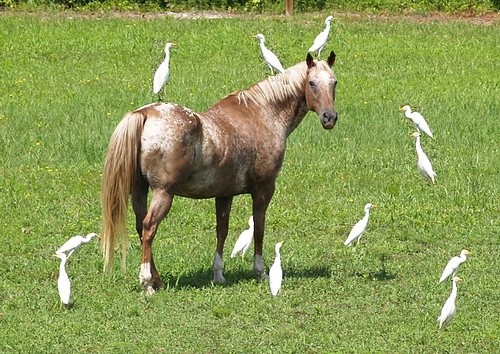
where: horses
[99,50,338,296]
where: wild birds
[344,203,378,248]
[438,249,473,285]
[153,43,177,103]
[230,215,254,264]
[307,16,338,61]
[436,276,462,330]
[51,252,71,310]
[250,33,285,76]
[410,132,438,185]
[55,233,101,260]
[269,240,285,295]
[399,105,433,138]
